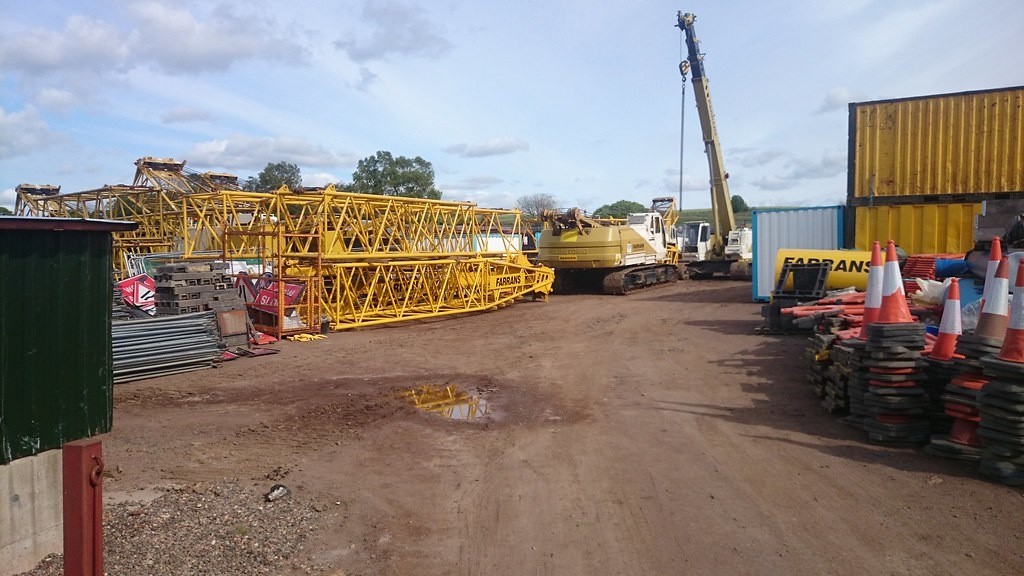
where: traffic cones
[831,235,1024,478]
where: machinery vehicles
[674,11,752,280]
[535,197,679,295]
